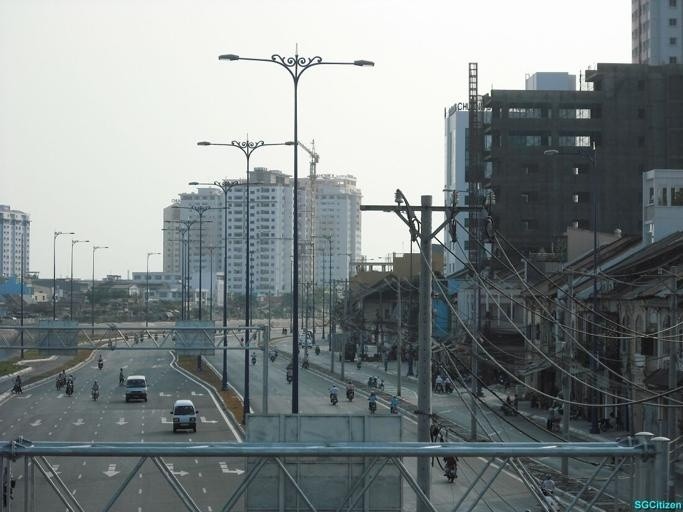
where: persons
[434,373,446,393]
[530,395,538,409]
[390,394,397,407]
[54,373,64,384]
[90,380,99,399]
[541,472,556,495]
[327,383,339,394]
[443,375,450,394]
[61,369,66,379]
[546,406,553,432]
[240,327,320,373]
[513,393,519,416]
[107,331,164,346]
[9,374,23,395]
[118,366,123,382]
[368,390,377,404]
[97,353,103,365]
[506,395,513,405]
[65,372,74,387]
[345,379,354,393]
[442,452,457,469]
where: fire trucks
[297,138,321,178]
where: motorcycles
[56,360,103,401]
[11,375,23,395]
[444,466,457,482]
[252,351,321,384]
[328,364,454,414]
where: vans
[170,399,199,432]
[126,375,147,401]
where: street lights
[52,231,75,320]
[190,180,265,326]
[162,205,231,321]
[218,42,375,417]
[91,247,109,333]
[70,239,91,322]
[198,129,293,424]
[146,252,161,327]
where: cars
[298,331,312,348]
